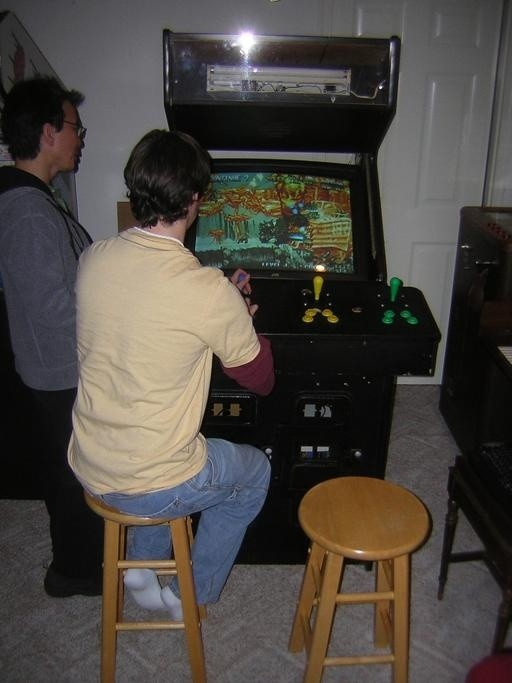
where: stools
[84,476,205,682]
[286,477,432,681]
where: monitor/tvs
[190,164,361,278]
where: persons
[69,128,275,623]
[1,73,105,598]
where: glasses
[63,117,87,137]
